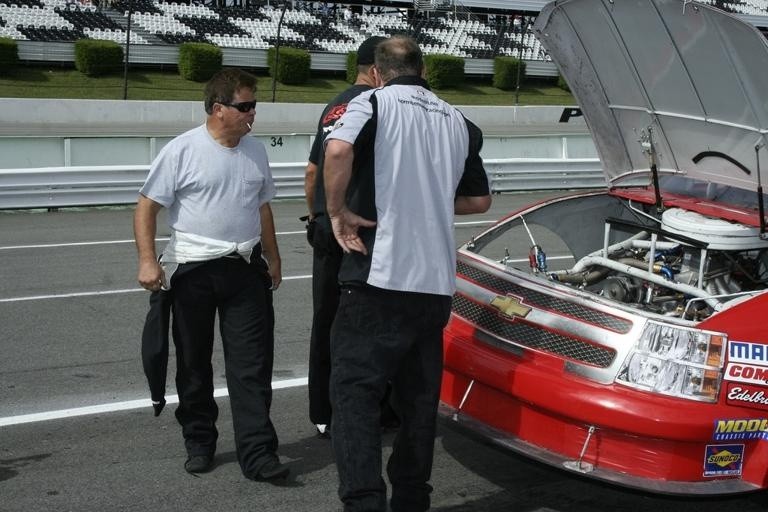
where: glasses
[206,100,257,112]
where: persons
[134,67,289,481]
[297,34,388,440]
[323,38,493,511]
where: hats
[357,37,387,64]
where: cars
[432,1,768,508]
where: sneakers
[317,422,332,439]
[185,455,211,472]
[259,461,290,479]
